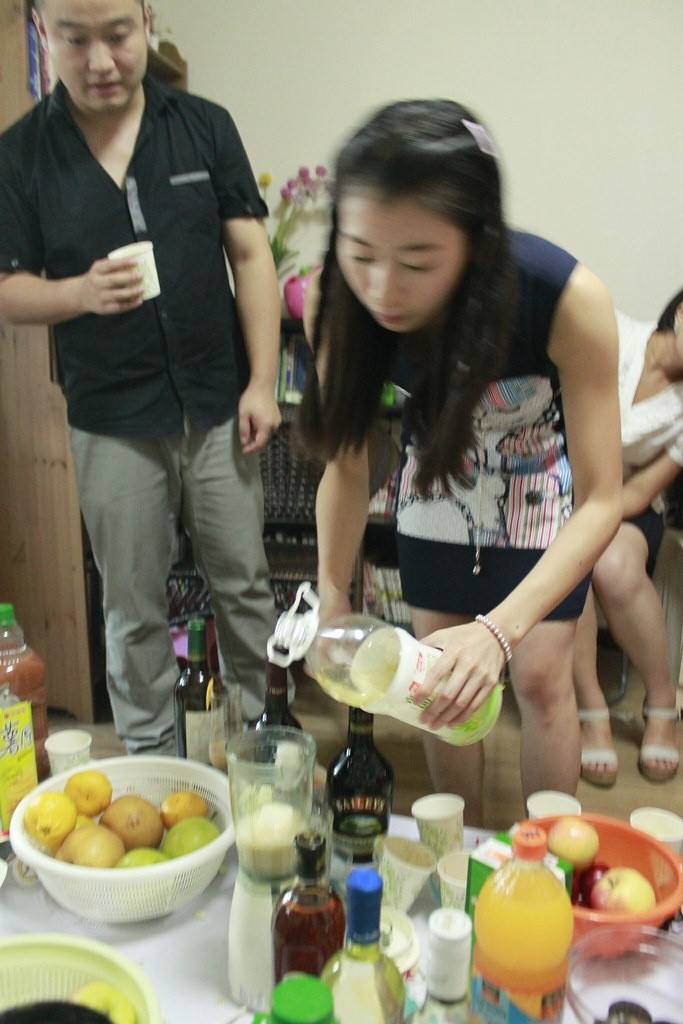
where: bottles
[465,824,575,1024]
[0,603,54,838]
[322,705,394,864]
[265,581,504,748]
[264,972,343,1024]
[173,618,230,774]
[321,865,407,1023]
[273,830,347,979]
[248,661,304,737]
[400,902,488,1024]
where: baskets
[0,935,162,1024]
[522,809,683,953]
[12,756,235,923]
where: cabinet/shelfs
[1,0,108,721]
[168,396,398,660]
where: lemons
[24,771,111,851]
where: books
[259,407,421,519]
[273,333,312,405]
[154,560,413,630]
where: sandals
[640,695,679,782]
[579,707,619,788]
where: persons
[0,0,303,768]
[302,98,625,829]
[569,288,683,787]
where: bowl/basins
[515,813,683,954]
[566,923,683,1023]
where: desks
[0,816,683,1023]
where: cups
[107,240,161,301]
[629,806,683,855]
[411,793,465,864]
[527,789,581,822]
[434,848,473,909]
[377,835,437,913]
[43,729,95,776]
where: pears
[56,791,220,870]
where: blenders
[225,724,335,1017]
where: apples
[545,815,658,915]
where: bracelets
[475,614,512,661]
